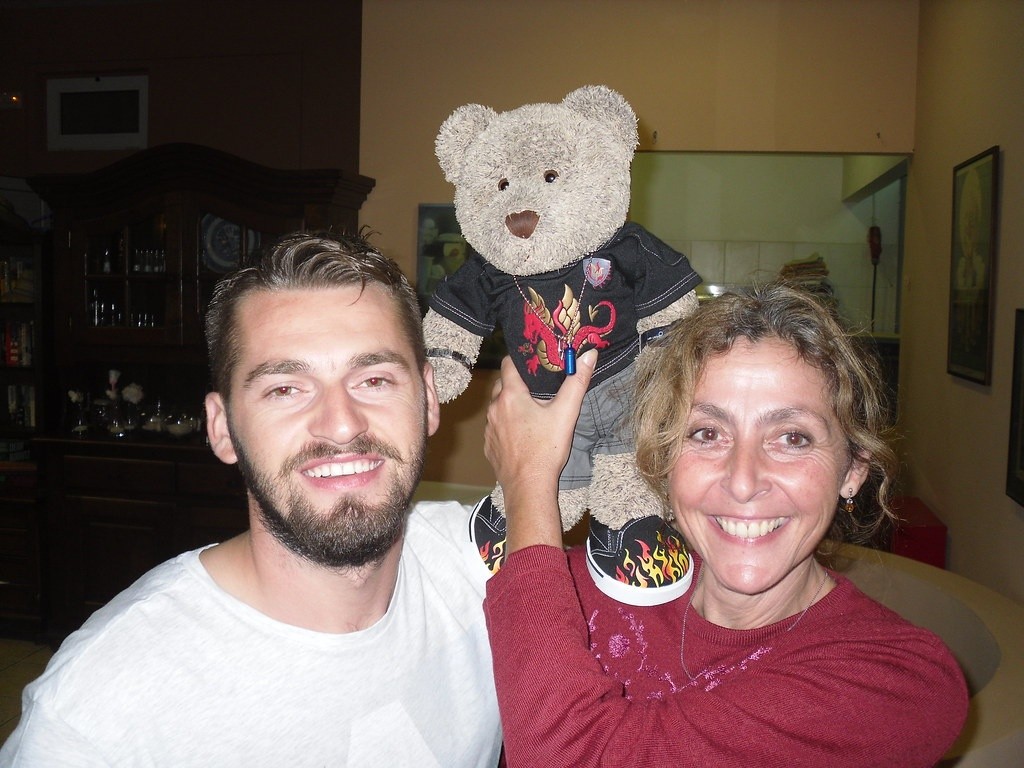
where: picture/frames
[417,203,509,370]
[946,146,1000,385]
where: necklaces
[681,569,827,679]
[513,252,594,376]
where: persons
[483,282,971,767]
[0,231,503,766]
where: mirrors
[629,148,911,430]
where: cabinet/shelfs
[27,143,382,644]
[0,197,48,643]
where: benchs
[411,481,1024,768]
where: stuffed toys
[423,85,706,607]
